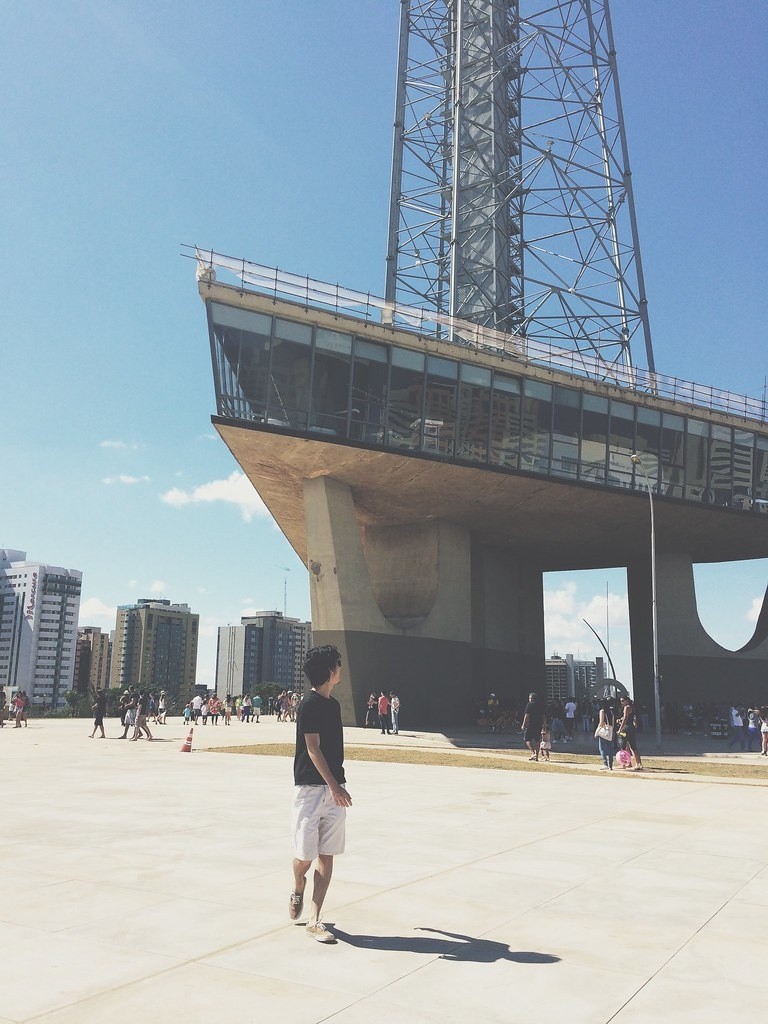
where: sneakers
[305,916,335,942]
[289,875,307,920]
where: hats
[124,690,129,695]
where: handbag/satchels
[596,722,612,741]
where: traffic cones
[180,728,194,752]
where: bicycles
[487,711,523,735]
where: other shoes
[99,735,105,738]
[88,735,94,738]
[529,755,538,761]
[138,733,143,738]
[147,736,152,741]
[601,766,612,770]
[131,738,137,740]
[119,735,126,739]
[631,765,643,770]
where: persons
[488,693,499,705]
[289,644,353,941]
[0,686,29,728]
[365,689,400,735]
[520,690,642,771]
[87,690,106,738]
[671,701,768,756]
[118,684,167,741]
[183,704,191,725]
[191,692,262,725]
[267,689,304,722]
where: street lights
[631,454,662,741]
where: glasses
[337,659,341,667]
